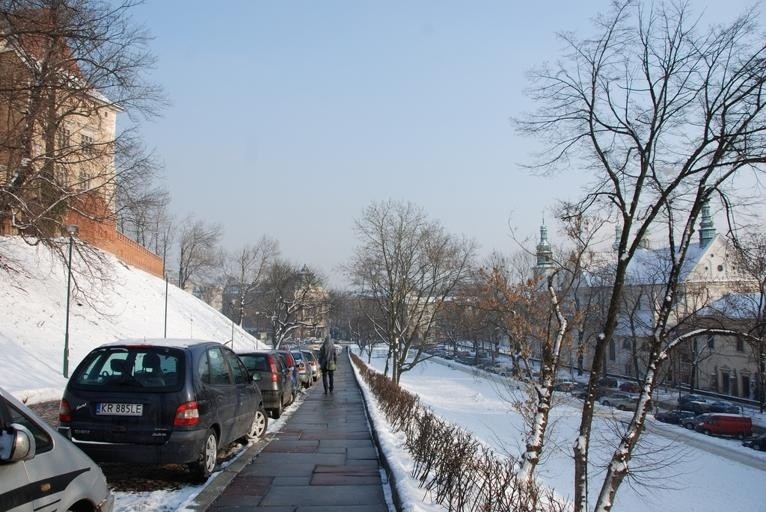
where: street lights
[63,225,77,379]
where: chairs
[99,359,126,384]
[140,353,166,386]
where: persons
[318,333,337,394]
